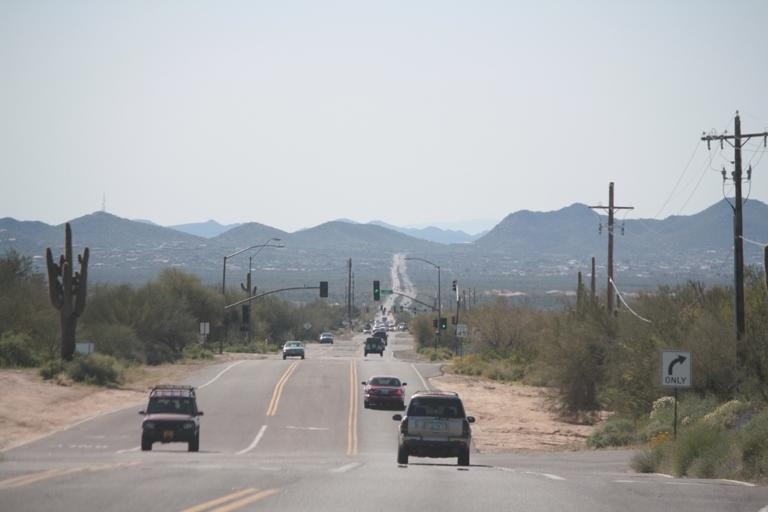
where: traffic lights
[439,317,447,329]
[373,280,379,301]
[452,280,457,292]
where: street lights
[248,237,281,345]
[222,243,285,296]
[404,257,441,315]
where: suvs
[138,385,204,452]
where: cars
[361,376,407,411]
[392,388,474,467]
[319,331,334,344]
[362,316,407,357]
[281,340,305,360]
